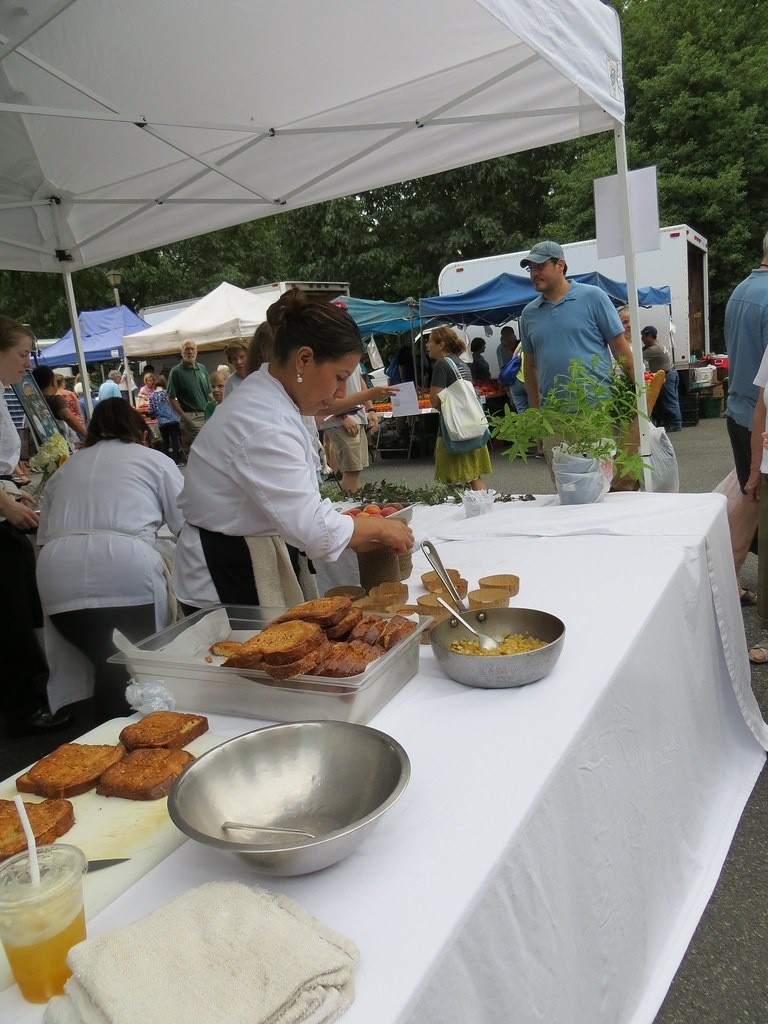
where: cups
[0,843,89,1005]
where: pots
[420,539,567,689]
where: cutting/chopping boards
[0,715,249,993]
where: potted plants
[485,356,649,504]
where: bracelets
[368,407,376,412]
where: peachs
[341,502,403,520]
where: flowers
[28,433,71,504]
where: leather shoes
[5,706,72,737]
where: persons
[469,304,683,460]
[725,230,768,662]
[173,288,413,631]
[426,326,493,492]
[32,338,249,464]
[32,336,401,489]
[519,241,644,491]
[36,398,184,728]
[0,314,41,701]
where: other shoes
[516,450,545,459]
[664,425,681,432]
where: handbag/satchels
[704,466,743,518]
[499,356,522,386]
[645,419,680,494]
[438,357,491,456]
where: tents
[0,0,651,494]
[331,297,448,397]
[418,271,674,390]
[122,282,276,409]
[29,304,152,368]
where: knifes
[86,857,132,873]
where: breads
[119,710,209,751]
[0,798,75,864]
[16,742,127,798]
[96,747,197,801]
[210,596,417,693]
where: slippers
[738,588,759,605]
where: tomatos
[474,379,498,395]
[364,399,432,413]
[645,371,654,383]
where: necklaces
[756,264,768,269]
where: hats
[520,241,564,268]
[641,325,657,335]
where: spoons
[435,596,499,650]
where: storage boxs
[695,356,728,408]
[105,594,435,723]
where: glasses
[526,261,554,273]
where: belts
[664,367,675,374]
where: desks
[0,488,767,1024]
[370,408,437,460]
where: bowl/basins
[168,719,412,879]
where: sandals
[747,641,768,663]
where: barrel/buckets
[694,367,713,386]
[710,354,728,369]
[701,395,723,418]
